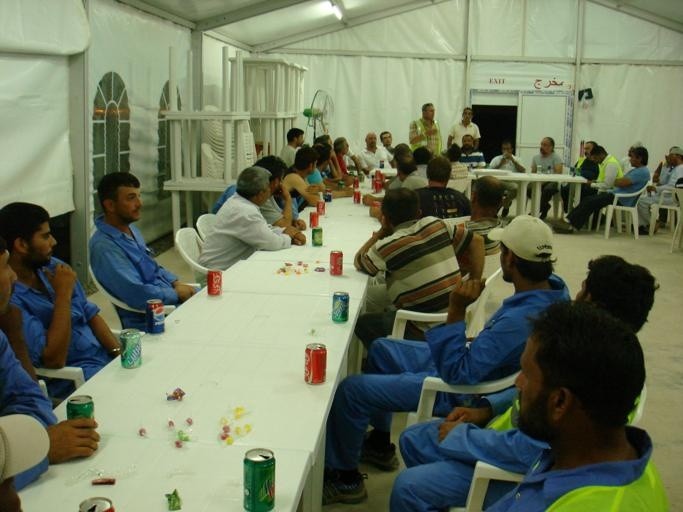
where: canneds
[146,299,164,334]
[78,496,116,511]
[537,165,543,175]
[330,250,343,276]
[120,329,142,370]
[67,395,94,430]
[309,160,385,246]
[207,270,222,296]
[332,292,350,324]
[305,343,327,385]
[244,449,275,511]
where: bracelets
[108,348,121,354]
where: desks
[163,47,308,250]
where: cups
[537,165,542,175]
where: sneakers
[639,219,661,235]
[322,470,368,505]
[554,217,574,234]
[360,432,399,471]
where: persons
[390,256,660,512]
[0,234,99,493]
[489,137,682,235]
[484,299,670,512]
[89,172,202,330]
[196,127,396,287]
[322,214,571,506]
[0,414,50,512]
[0,202,121,402]
[356,103,513,351]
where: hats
[0,413,50,483]
[488,214,555,262]
